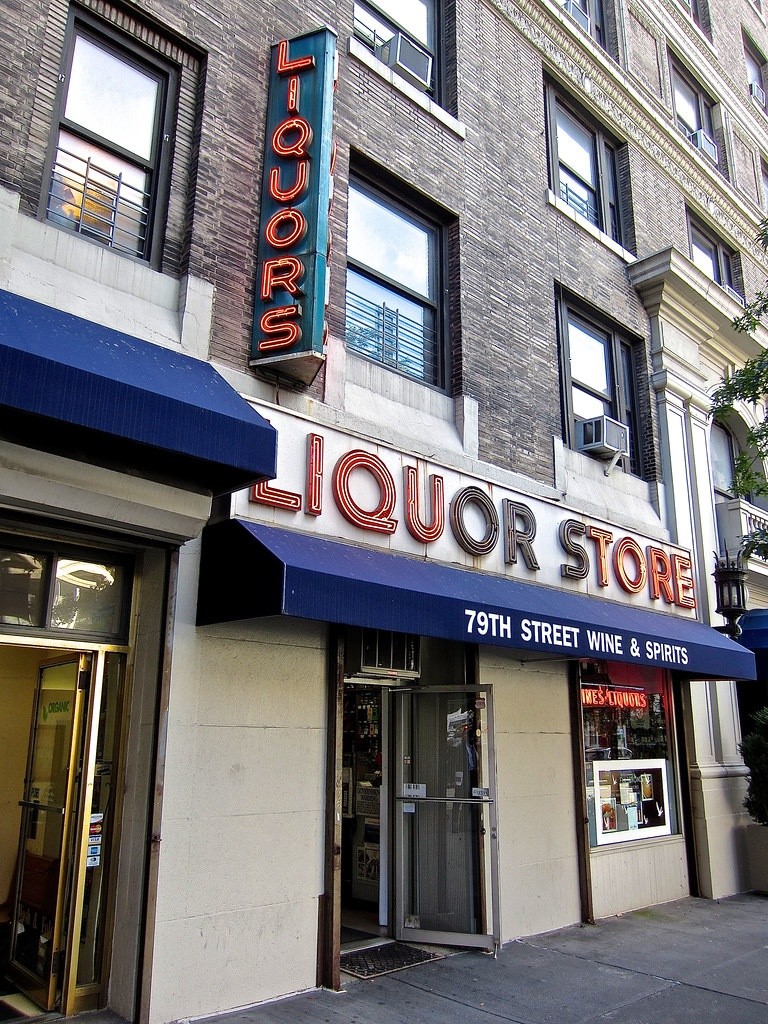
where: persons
[452,729,476,833]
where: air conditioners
[576,416,629,459]
[687,129,718,166]
[374,32,432,92]
[749,81,765,108]
[344,627,422,681]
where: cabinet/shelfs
[355,693,382,741]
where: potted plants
[736,707,768,892]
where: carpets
[340,943,446,979]
[0,999,30,1024]
[340,925,380,943]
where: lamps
[521,657,608,674]
[710,537,750,641]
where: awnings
[734,609,768,648]
[196,516,756,682]
[0,289,277,497]
